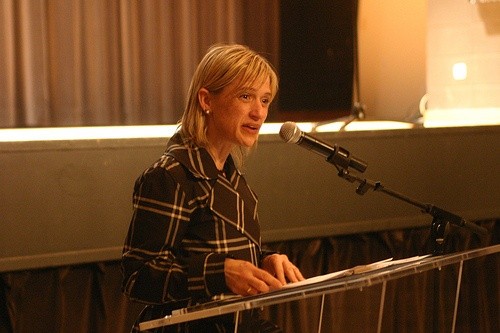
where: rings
[246,287,252,293]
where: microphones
[279,121,368,173]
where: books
[282,255,438,290]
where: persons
[121,41,305,333]
